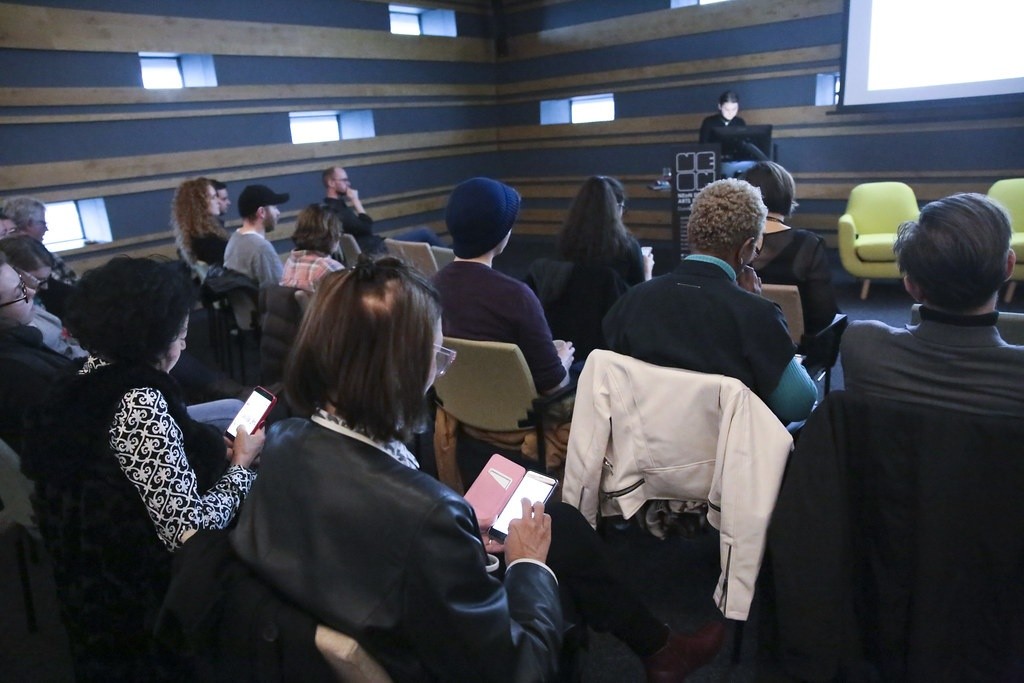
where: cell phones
[488,468,559,541]
[223,385,277,442]
[641,247,653,256]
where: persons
[19,253,267,560]
[170,177,231,266]
[423,177,578,477]
[736,162,841,340]
[700,91,746,145]
[0,196,225,405]
[599,178,817,428]
[837,191,1024,417]
[223,255,670,683]
[224,185,290,283]
[555,175,654,290]
[321,165,450,254]
[279,202,347,295]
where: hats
[237,185,290,217]
[446,177,521,260]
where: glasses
[16,268,53,290]
[432,342,456,378]
[0,274,30,308]
[617,202,628,216]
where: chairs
[838,182,920,299]
[985,177,1024,303]
[0,237,848,683]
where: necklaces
[766,216,783,223]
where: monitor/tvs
[712,123,774,161]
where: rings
[488,540,492,544]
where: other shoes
[648,621,726,683]
